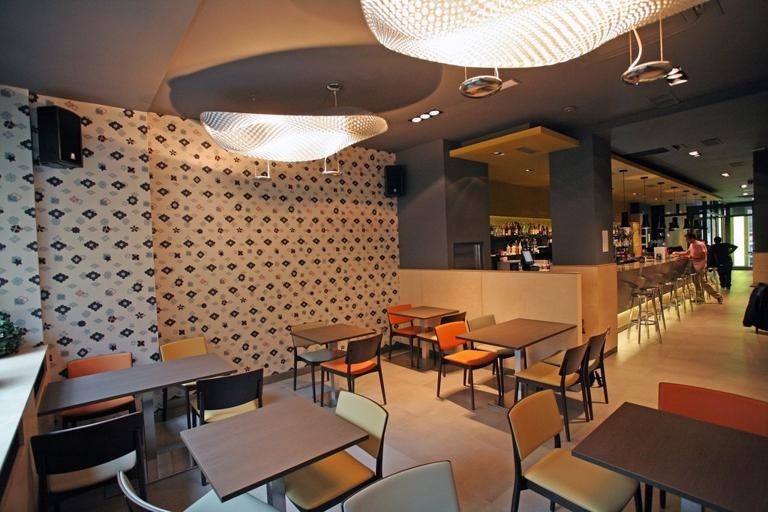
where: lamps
[702,196,708,229]
[359,0,702,98]
[200,83,388,179]
[641,177,652,230]
[657,182,666,230]
[692,193,703,229]
[671,186,680,229]
[683,191,692,229]
[620,170,631,227]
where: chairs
[434,321,502,410]
[341,461,460,512]
[319,334,387,407]
[416,311,466,377]
[513,342,590,440]
[160,337,207,421]
[197,367,264,486]
[468,315,515,384]
[119,471,281,512]
[507,390,643,512]
[30,411,149,512]
[543,327,609,421]
[290,321,346,401]
[284,389,389,512]
[386,304,432,368]
[60,352,140,442]
[658,381,768,439]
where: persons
[707,236,738,295]
[672,233,723,305]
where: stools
[653,281,676,331]
[628,287,667,344]
[689,273,697,302]
[707,267,721,302]
[670,277,693,321]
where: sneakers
[717,296,724,304]
[691,299,707,304]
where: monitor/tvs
[521,250,534,267]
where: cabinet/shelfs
[0,344,51,512]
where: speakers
[382,165,407,197]
[35,105,83,170]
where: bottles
[495,239,539,254]
[614,225,632,258]
[490,220,546,236]
